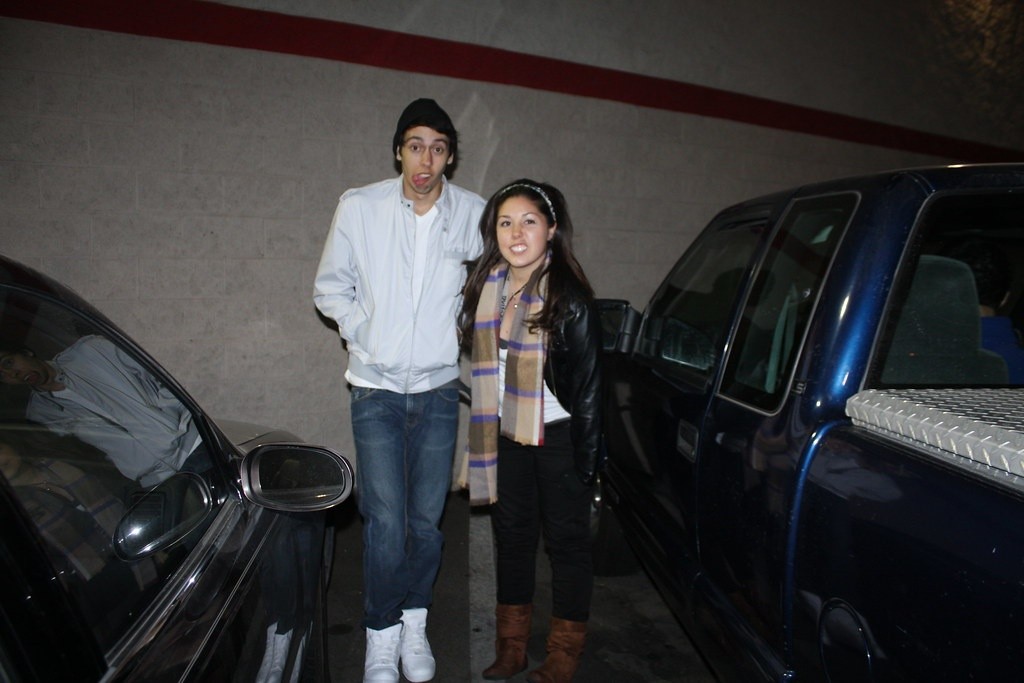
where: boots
[529,615,589,683]
[401,608,436,682]
[256,621,307,683]
[481,602,533,680]
[363,624,402,683]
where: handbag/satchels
[580,458,631,579]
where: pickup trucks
[592,160,1024,682]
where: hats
[395,96,456,145]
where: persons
[17,332,217,509]
[452,178,606,683]
[310,95,493,682]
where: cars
[0,253,357,682]
